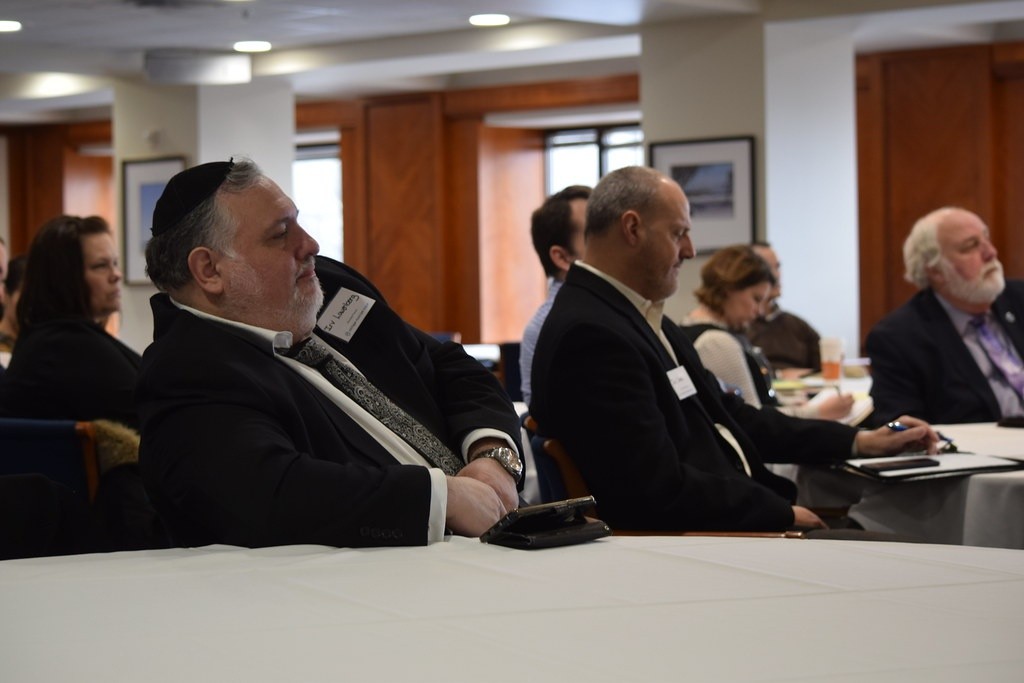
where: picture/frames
[646,133,757,255]
[118,154,184,285]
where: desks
[0,420,1024,683]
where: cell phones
[860,458,940,472]
[478,496,597,543]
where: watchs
[469,447,525,485]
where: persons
[678,243,867,531]
[734,241,824,400]
[860,206,1024,430]
[514,186,746,423]
[121,157,531,563]
[0,216,146,563]
[530,167,939,535]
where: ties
[968,312,1024,398]
[274,336,466,476]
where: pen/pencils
[835,384,842,396]
[886,422,949,442]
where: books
[773,370,874,428]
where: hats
[150,157,235,237]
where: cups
[819,337,841,380]
